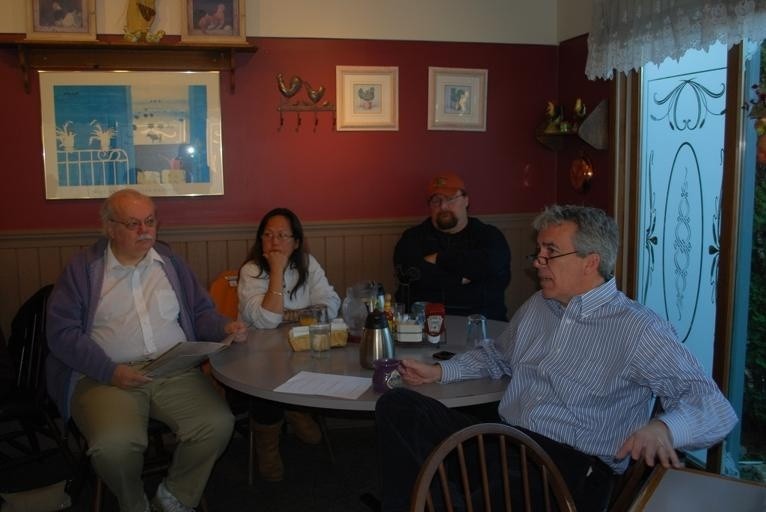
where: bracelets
[266,288,285,299]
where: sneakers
[284,409,323,444]
[153,481,195,512]
[253,419,284,481]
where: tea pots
[359,308,395,370]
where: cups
[372,357,408,393]
[299,310,318,326]
[465,314,488,351]
[309,323,331,361]
[391,303,405,323]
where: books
[136,327,239,382]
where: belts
[508,425,611,480]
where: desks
[625,463,765,512]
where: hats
[426,173,466,199]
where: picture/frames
[180,1,245,45]
[336,65,399,131]
[428,66,488,133]
[37,67,226,199]
[24,0,95,42]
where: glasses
[429,193,465,206]
[109,216,157,231]
[260,232,294,240]
[525,251,576,265]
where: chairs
[29,285,211,512]
[494,393,660,511]
[413,423,579,511]
[207,267,338,484]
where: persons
[43,188,248,511]
[392,170,515,322]
[373,203,739,511]
[237,206,343,484]
